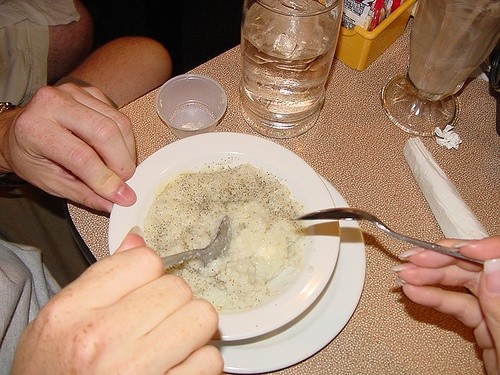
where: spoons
[289,208,485,270]
[150,215,235,273]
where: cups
[240,0,343,139]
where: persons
[391,236,500,374]
[0,0,173,109]
[0,82,224,375]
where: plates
[203,174,366,373]
[108,130,341,344]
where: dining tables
[67,16,500,375]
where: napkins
[403,136,490,240]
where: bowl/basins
[155,73,229,140]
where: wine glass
[379,0,500,138]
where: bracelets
[0,101,16,113]
